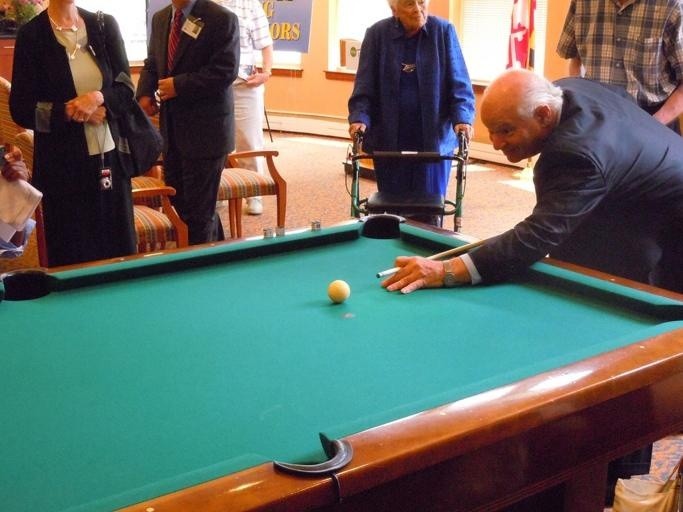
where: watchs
[443,258,457,287]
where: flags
[505,0,537,71]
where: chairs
[0,77,23,150]
[15,127,186,268]
[124,65,289,235]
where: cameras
[98,167,113,192]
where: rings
[77,119,84,123]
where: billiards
[329,280,350,303]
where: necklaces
[48,9,81,59]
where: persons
[556,0,683,135]
[347,0,476,227]
[214,0,274,216]
[377,66,683,512]
[135,0,240,246]
[7,1,163,268]
[0,142,37,259]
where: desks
[1,210,681,512]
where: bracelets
[263,68,272,76]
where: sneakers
[246,199,262,214]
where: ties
[167,8,183,77]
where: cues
[376,229,512,279]
